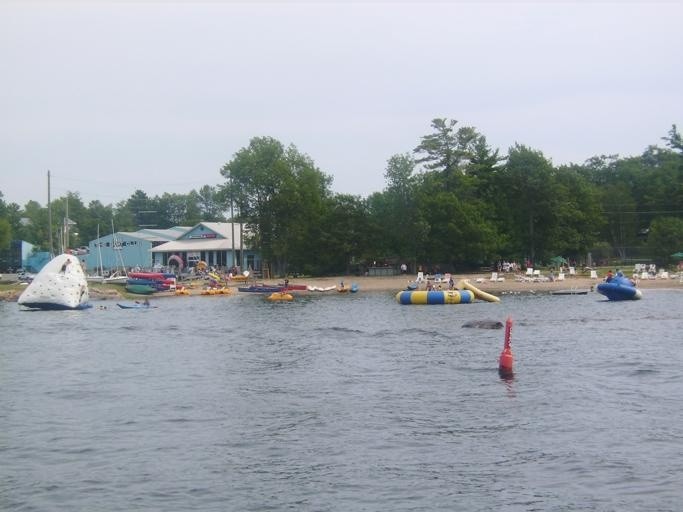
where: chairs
[410,264,679,287]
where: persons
[648,264,656,275]
[606,270,614,283]
[196,264,236,291]
[630,274,640,287]
[58,258,72,274]
[549,269,555,282]
[616,268,624,277]
[284,278,289,291]
[417,277,454,291]
[81,259,86,272]
[497,259,531,274]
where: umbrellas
[669,251,683,259]
[550,256,568,269]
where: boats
[394,282,474,303]
[267,292,293,299]
[200,272,249,295]
[595,276,642,302]
[124,269,178,296]
[237,280,362,294]
[175,287,190,296]
[116,302,159,310]
[461,280,501,302]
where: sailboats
[37,217,135,286]
